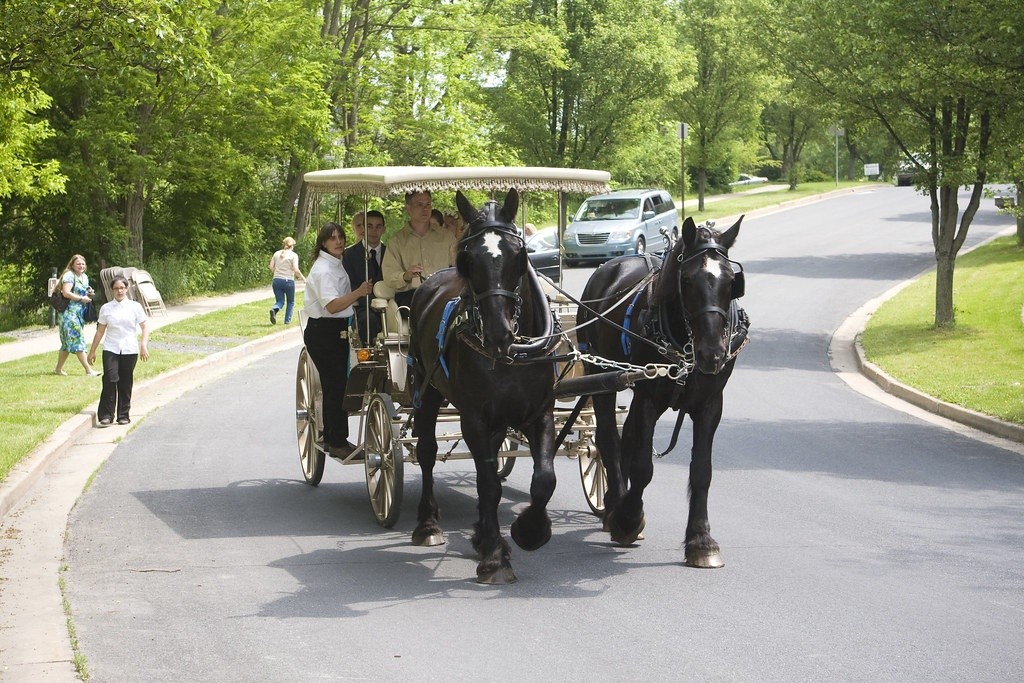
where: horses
[575,213,751,568]
[406,186,557,584]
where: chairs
[373,281,408,335]
[100,266,167,317]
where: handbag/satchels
[49,270,75,312]
[84,298,98,322]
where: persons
[86,277,149,427]
[54,255,102,376]
[430,209,464,239]
[343,210,387,348]
[526,223,554,247]
[269,237,306,325]
[380,189,457,319]
[300,221,373,460]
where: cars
[897,152,942,186]
[727,173,769,186]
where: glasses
[113,287,126,291]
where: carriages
[294,164,752,586]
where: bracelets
[78,297,82,302]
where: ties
[370,249,378,281]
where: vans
[563,186,681,268]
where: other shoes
[329,445,365,460]
[100,418,111,424]
[55,370,67,376]
[118,418,129,424]
[270,309,277,325]
[323,443,329,452]
[86,371,102,377]
